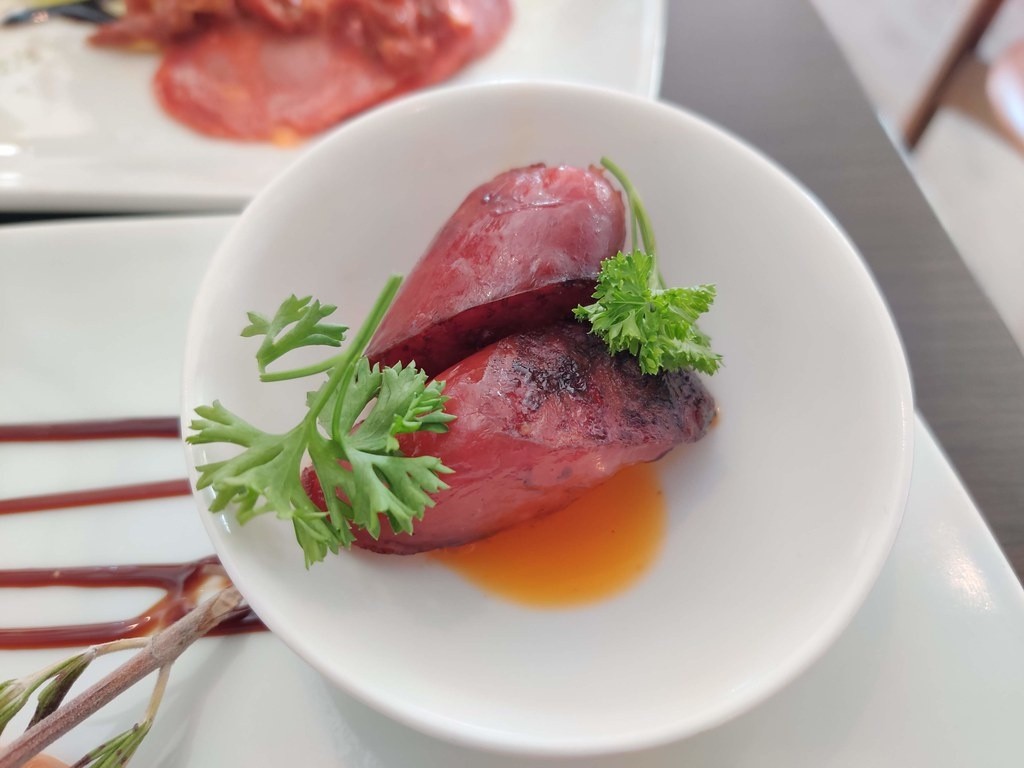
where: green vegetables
[572,158,725,376]
[183,272,456,568]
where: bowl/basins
[176,75,916,758]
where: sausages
[353,162,625,380]
[301,321,718,559]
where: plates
[0,211,1024,768]
[0,2,673,210]
